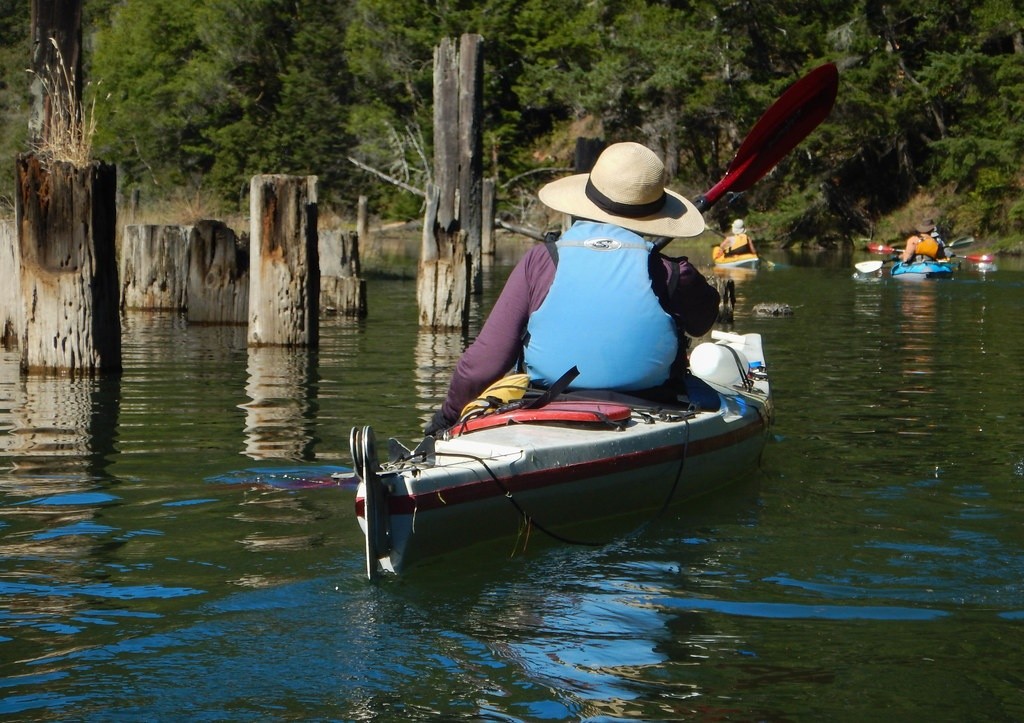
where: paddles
[867,241,997,264]
[854,235,975,274]
[704,224,776,268]
[654,58,841,254]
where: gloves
[422,415,448,438]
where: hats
[914,217,934,233]
[539,141,704,239]
[732,219,745,235]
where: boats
[712,244,762,269]
[350,330,774,583]
[890,262,955,282]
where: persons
[719,219,758,258]
[892,217,956,263]
[423,143,721,437]
